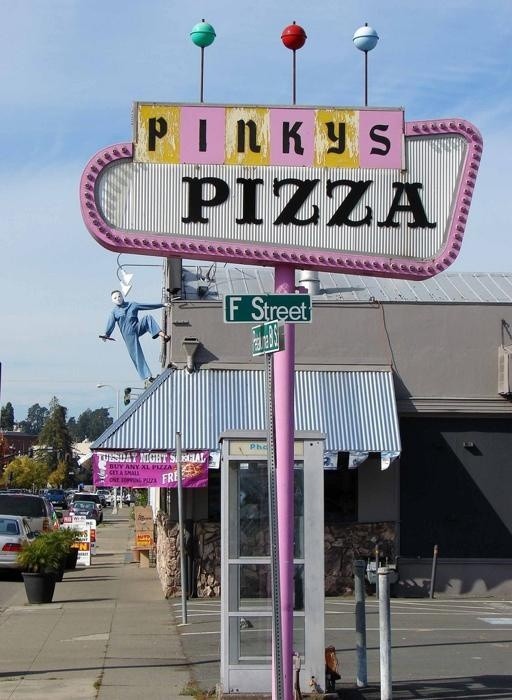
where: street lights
[96,382,124,514]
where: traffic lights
[65,452,71,463]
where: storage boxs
[134,504,155,559]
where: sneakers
[160,333,170,344]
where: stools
[132,547,153,568]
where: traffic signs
[249,317,281,358]
[221,291,316,325]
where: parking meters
[350,558,370,689]
[366,557,401,699]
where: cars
[0,514,38,571]
[0,485,131,540]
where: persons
[101,289,172,388]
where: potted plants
[45,531,70,582]
[14,536,63,605]
[63,530,82,571]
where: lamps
[116,264,162,296]
[115,248,135,282]
